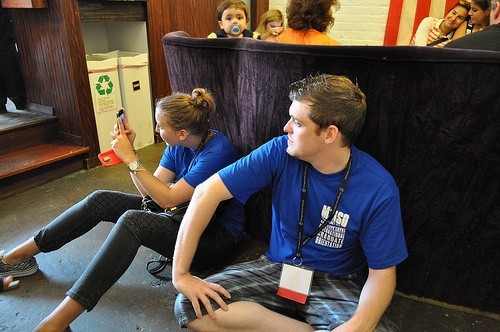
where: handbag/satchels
[141,194,230,233]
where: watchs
[127,160,143,173]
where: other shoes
[0,250,38,278]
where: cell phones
[117,109,125,130]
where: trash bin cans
[84,49,156,152]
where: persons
[410,0,471,47]
[207,0,284,40]
[468,0,492,33]
[442,0,500,52]
[253,9,284,36]
[265,0,341,45]
[0,88,247,332]
[171,75,409,332]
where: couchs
[161,31,500,320]
